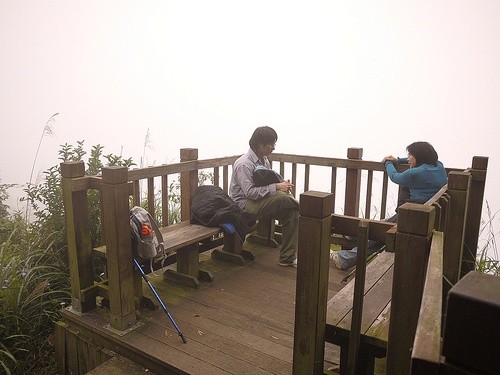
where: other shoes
[278,257,297,268]
[329,249,342,270]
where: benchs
[325,250,395,368]
[95,219,265,307]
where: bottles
[141,226,153,240]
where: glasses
[262,141,277,147]
[407,152,412,157]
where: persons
[329,142,447,270]
[229,126,299,268]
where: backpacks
[129,206,166,261]
